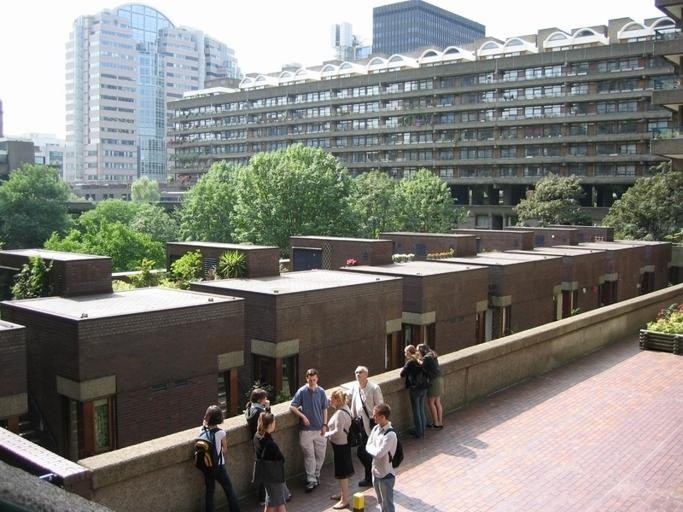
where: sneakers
[305,478,319,494]
[358,477,373,487]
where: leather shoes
[330,493,350,510]
[408,423,443,439]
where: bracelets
[323,423,327,428]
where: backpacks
[192,425,223,475]
[336,408,367,447]
[384,427,404,468]
[407,357,432,390]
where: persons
[323,390,363,509]
[288,369,330,493]
[415,344,444,429]
[401,345,431,439]
[365,404,404,512]
[345,365,385,487]
[245,389,292,504]
[193,405,242,512]
[250,413,288,512]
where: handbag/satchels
[369,417,377,430]
